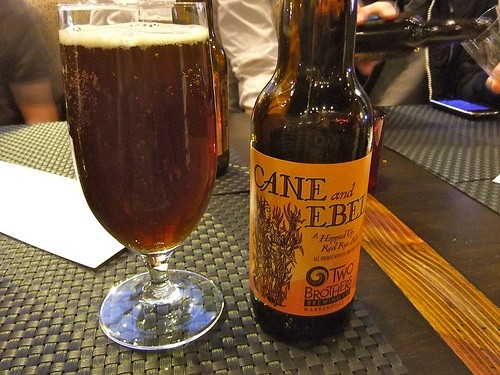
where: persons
[85,0,500,116]
[0,0,65,127]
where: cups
[368,109,388,191]
[462,5,500,75]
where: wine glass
[58,6,225,348]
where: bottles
[173,0,229,178]
[249,0,373,343]
[355,16,491,64]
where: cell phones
[429,98,500,120]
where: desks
[0,98,500,375]
